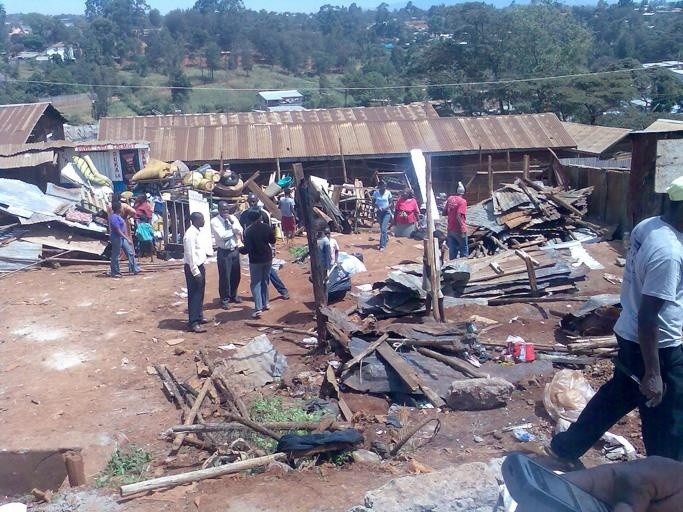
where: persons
[239,192,290,299]
[324,224,340,283]
[238,205,277,318]
[442,179,469,259]
[372,181,393,251]
[183,211,214,333]
[394,187,421,232]
[307,216,332,320]
[105,190,155,278]
[210,200,245,306]
[513,453,683,512]
[548,175,683,464]
[277,188,297,241]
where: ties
[225,220,232,250]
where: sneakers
[251,303,271,318]
[220,298,243,310]
[282,291,289,300]
[543,442,587,472]
[187,317,209,333]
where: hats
[456,181,465,195]
[665,175,683,201]
